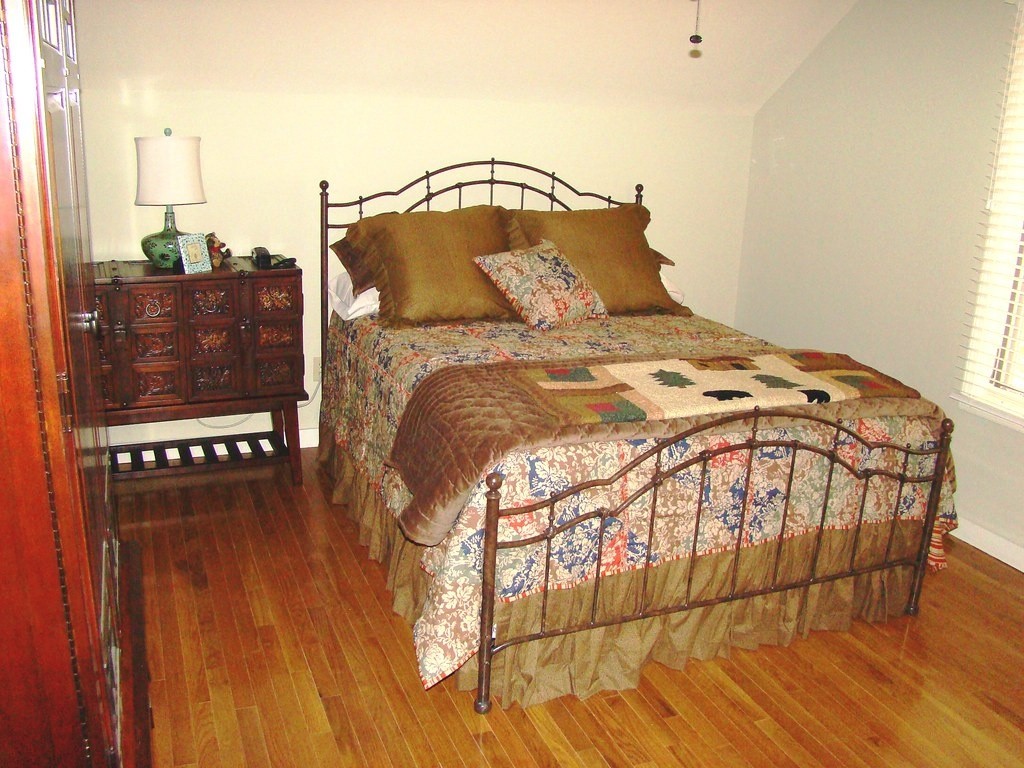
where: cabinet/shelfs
[2,0,155,768]
[93,254,309,488]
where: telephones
[251,247,298,270]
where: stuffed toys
[206,231,232,268]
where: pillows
[328,266,381,322]
[472,235,610,336]
[345,205,529,330]
[504,203,695,319]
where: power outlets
[313,357,322,382]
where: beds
[319,157,958,715]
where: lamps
[133,127,209,270]
